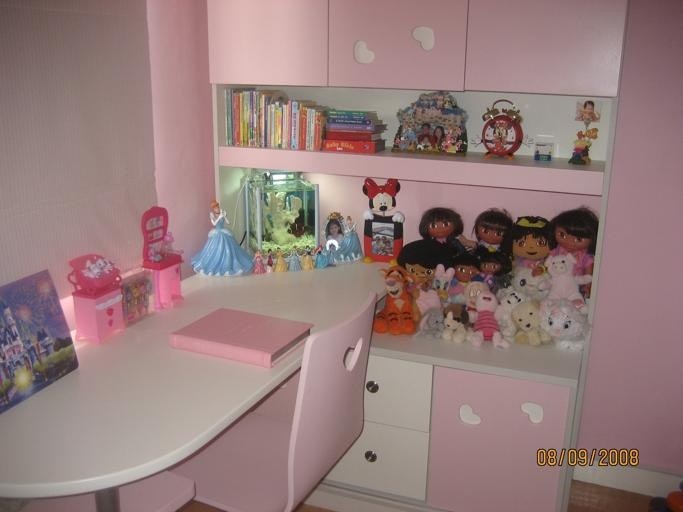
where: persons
[432,126,445,145]
[190,198,362,276]
[576,100,599,120]
[374,234,394,254]
[418,123,433,145]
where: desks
[1,255,393,512]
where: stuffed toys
[373,208,598,353]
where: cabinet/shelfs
[146,1,630,512]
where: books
[168,308,313,369]
[223,88,387,155]
